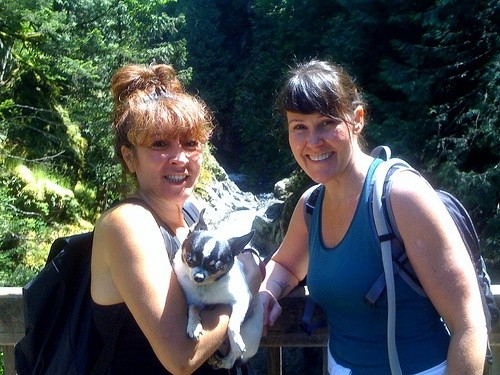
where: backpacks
[302,145,500,374]
[13,197,207,375]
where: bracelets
[242,248,266,283]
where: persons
[89,63,268,375]
[258,58,489,375]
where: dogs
[174,208,266,368]
